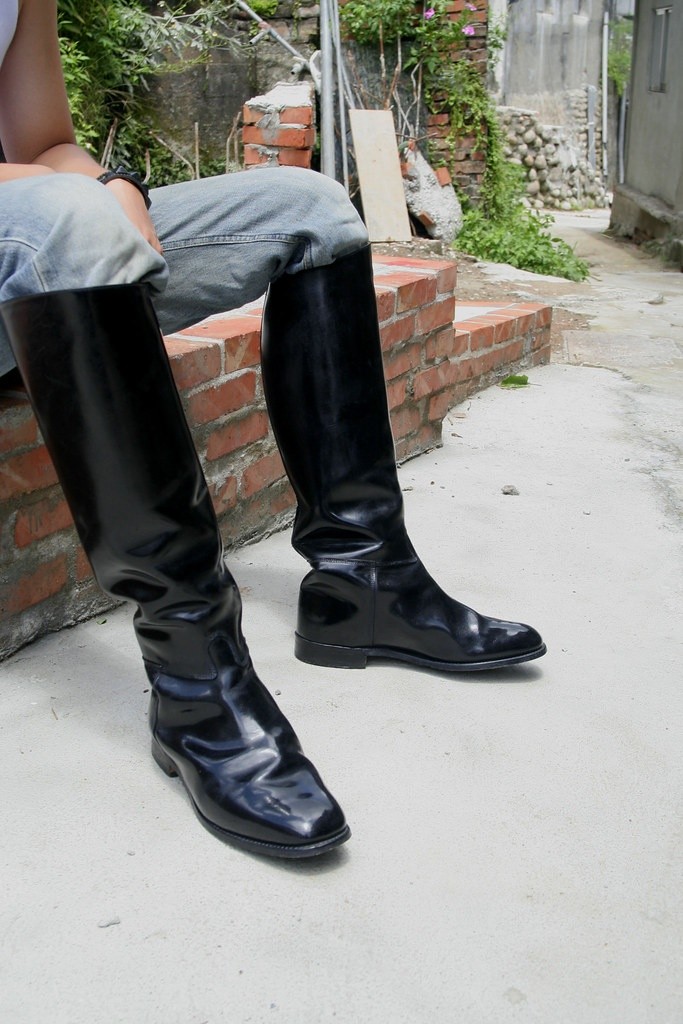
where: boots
[258,241,549,675]
[0,283,352,862]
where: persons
[0,0,547,858]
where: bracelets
[97,165,153,211]
